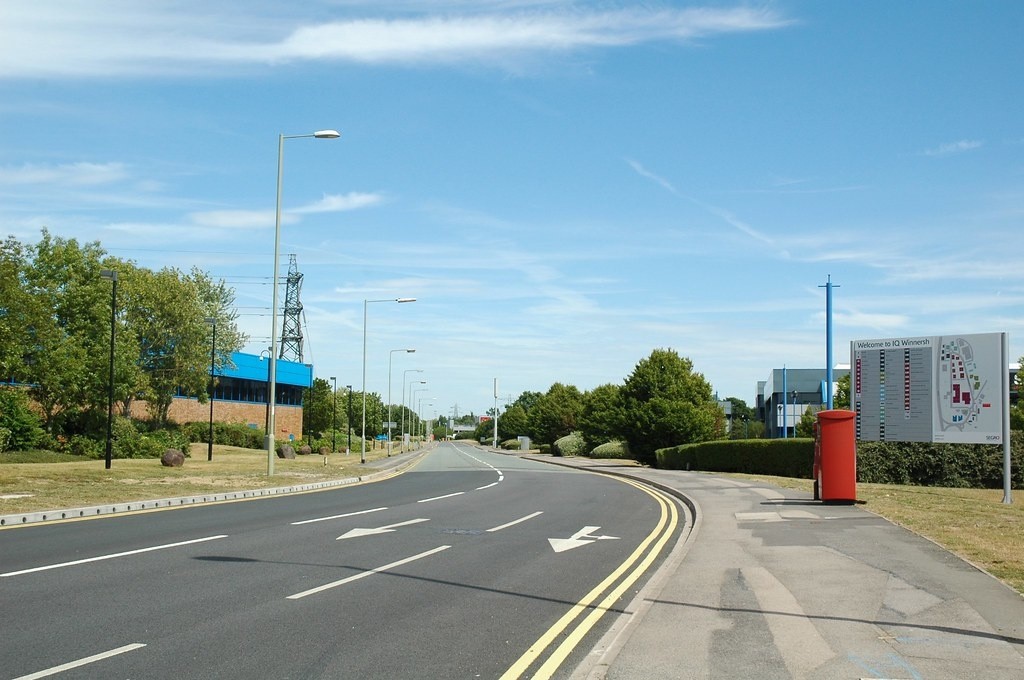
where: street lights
[265,128,340,482]
[329,377,337,454]
[401,369,425,452]
[259,349,270,450]
[359,297,418,463]
[305,362,312,446]
[346,384,353,454]
[203,317,219,461]
[412,388,428,450]
[386,348,418,458]
[99,269,119,469]
[418,396,438,446]
